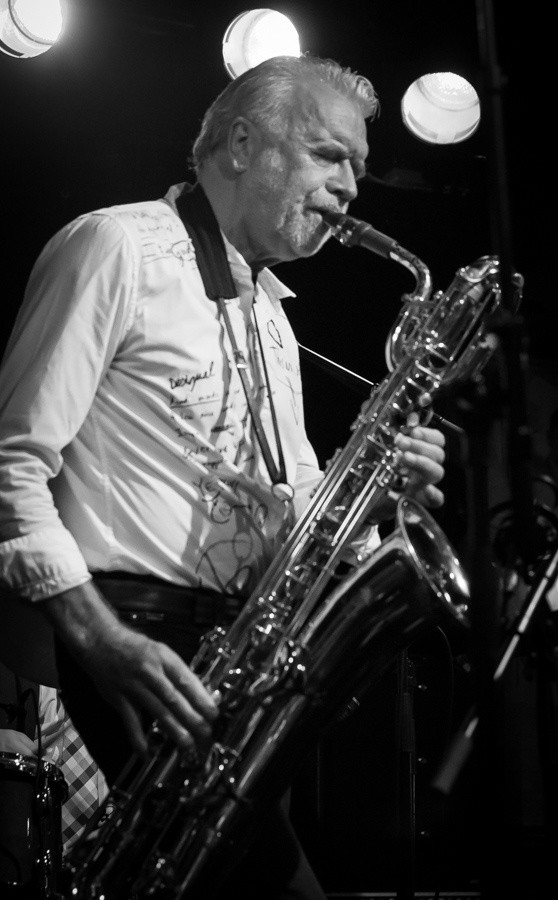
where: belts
[96,579,243,628]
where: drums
[0,748,70,900]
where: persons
[1,668,112,852]
[1,54,447,900]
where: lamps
[0,1,480,146]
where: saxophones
[59,207,510,898]
